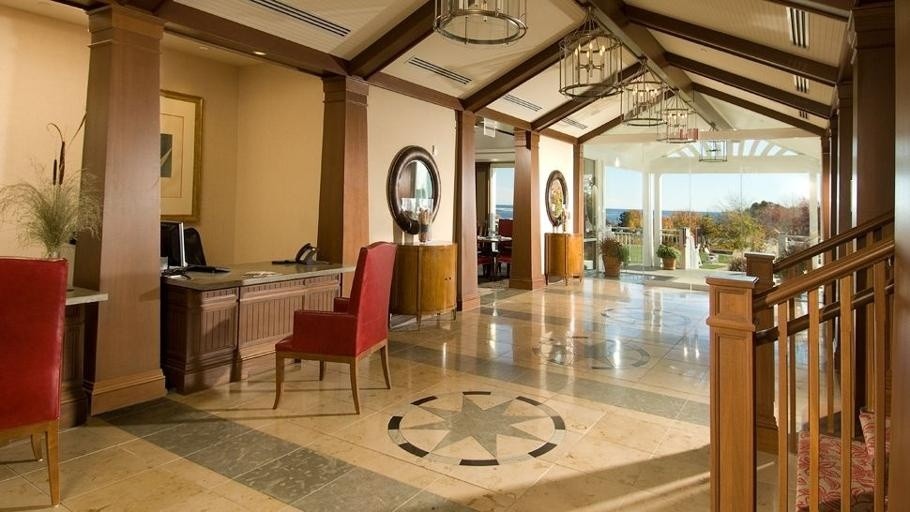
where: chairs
[273,242,398,414]
[477,219,513,281]
[0,256,70,506]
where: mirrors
[388,145,441,234]
[545,170,569,226]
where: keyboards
[188,264,229,273]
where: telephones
[272,243,319,265]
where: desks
[57,284,109,430]
[545,233,584,287]
[161,261,357,395]
[390,243,459,330]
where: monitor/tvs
[160,220,184,272]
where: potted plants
[600,237,629,277]
[656,245,681,270]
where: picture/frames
[160,89,204,222]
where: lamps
[699,124,727,162]
[656,91,698,144]
[433,0,528,50]
[559,8,623,98]
[621,57,668,126]
